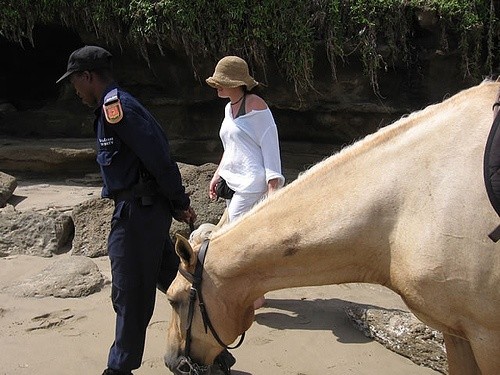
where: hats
[55,46,113,84]
[205,56,259,91]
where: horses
[163,75,499,375]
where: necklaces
[232,95,244,105]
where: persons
[56,45,236,375]
[207,56,285,311]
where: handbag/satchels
[213,177,235,200]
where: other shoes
[101,369,134,375]
